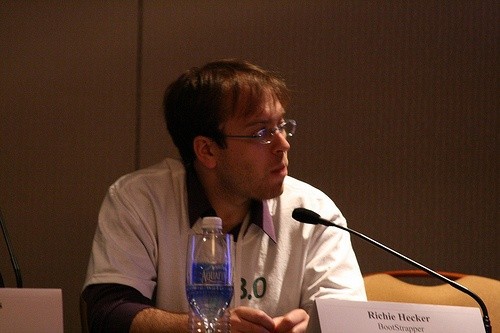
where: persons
[79,59,368,333]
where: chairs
[364,269,500,333]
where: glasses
[225,119,297,145]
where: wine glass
[185,233,236,333]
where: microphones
[292,208,492,333]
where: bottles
[188,216,232,333]
